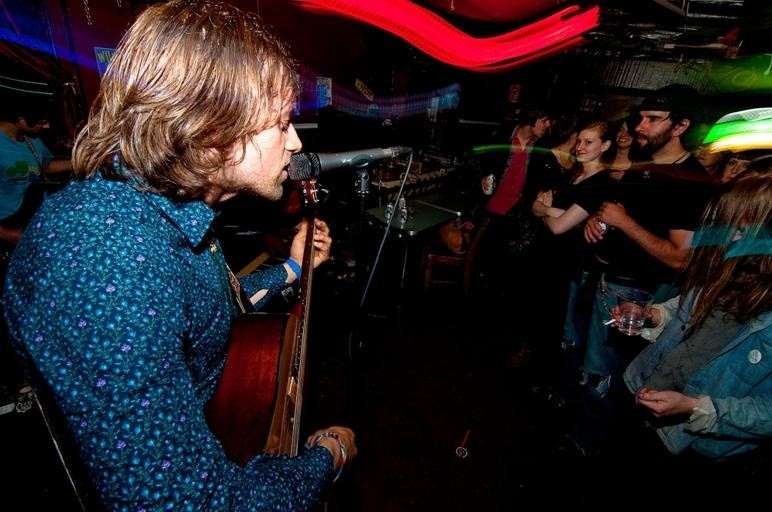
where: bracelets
[284,256,303,282]
[310,430,349,474]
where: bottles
[481,158,497,196]
[594,194,620,238]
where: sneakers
[560,339,576,353]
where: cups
[615,289,656,335]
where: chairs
[415,216,491,296]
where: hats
[627,84,704,120]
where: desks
[367,200,462,288]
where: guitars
[203,178,329,469]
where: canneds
[400,207,407,224]
[399,199,407,206]
[385,203,393,219]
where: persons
[465,75,770,511]
[2,0,366,512]
[1,91,74,244]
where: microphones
[287,146,410,183]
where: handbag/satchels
[441,218,474,255]
